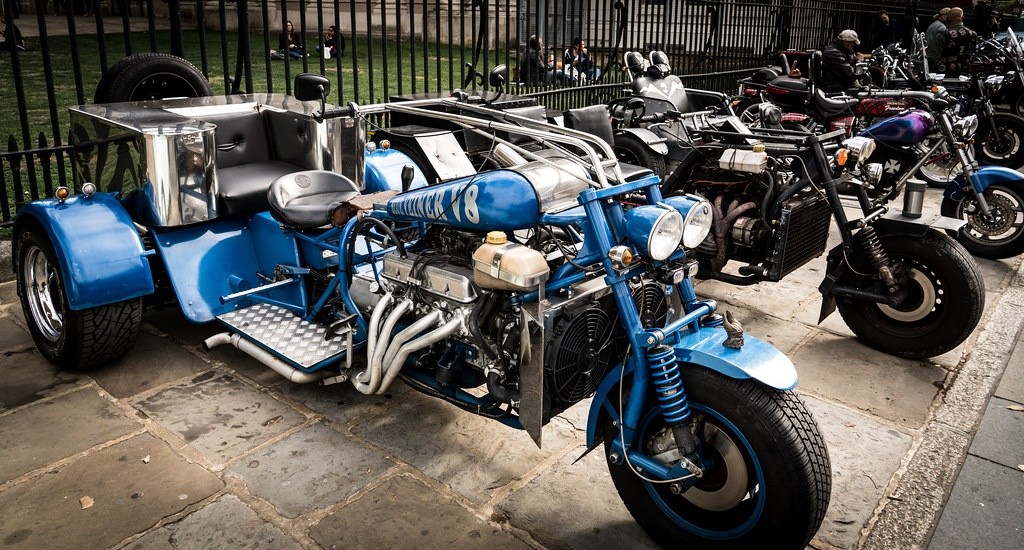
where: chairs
[192,110,302,216]
[502,105,549,156]
[811,50,846,97]
[562,104,654,185]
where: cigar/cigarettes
[852,50,855,54]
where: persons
[271,21,310,61]
[822,7,1000,95]
[0,14,24,53]
[518,35,601,88]
[315,25,345,58]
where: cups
[902,179,929,217]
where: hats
[837,29,861,45]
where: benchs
[624,49,728,142]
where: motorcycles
[8,0,1022,550]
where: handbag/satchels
[324,44,333,59]
[271,54,290,59]
